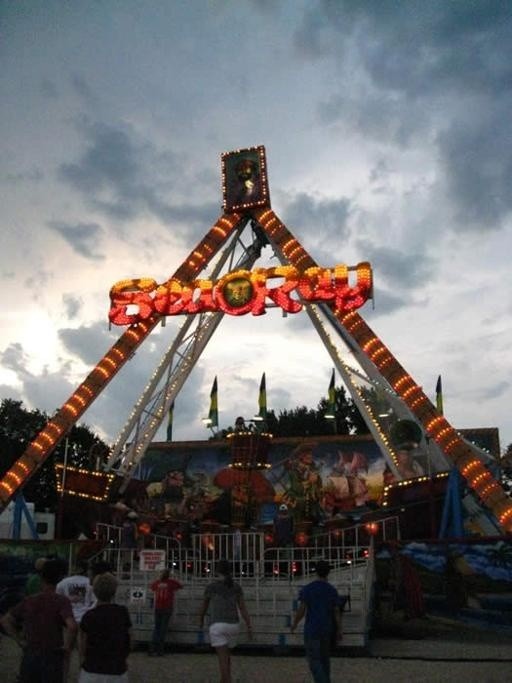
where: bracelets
[246,624,252,627]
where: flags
[325,370,335,415]
[435,376,442,415]
[168,401,174,439]
[258,373,266,419]
[210,378,217,425]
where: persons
[198,557,253,683]
[285,443,327,524]
[290,559,345,682]
[1,551,134,682]
[147,568,182,656]
[272,504,297,559]
